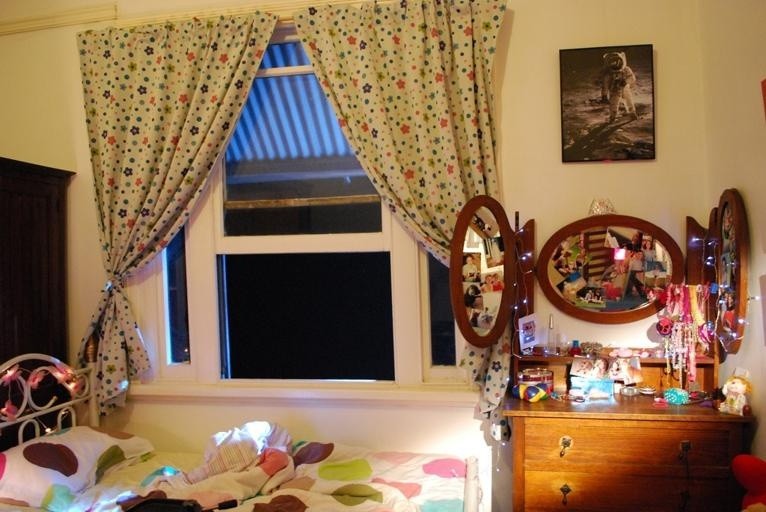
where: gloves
[620,81,626,86]
[601,95,609,103]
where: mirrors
[504,212,535,354]
[685,189,748,356]
[536,213,685,325]
[450,190,515,348]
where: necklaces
[660,282,711,387]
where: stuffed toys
[732,453,766,512]
[719,374,754,415]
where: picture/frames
[558,43,655,163]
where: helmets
[601,51,626,73]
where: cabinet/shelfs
[0,154,76,448]
[503,211,752,512]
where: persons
[598,61,613,106]
[589,356,610,377]
[491,273,504,291]
[629,249,645,273]
[480,274,493,292]
[598,52,638,123]
[642,240,655,262]
[461,254,479,281]
[717,292,736,328]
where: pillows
[0,425,154,510]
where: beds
[0,353,478,512]
